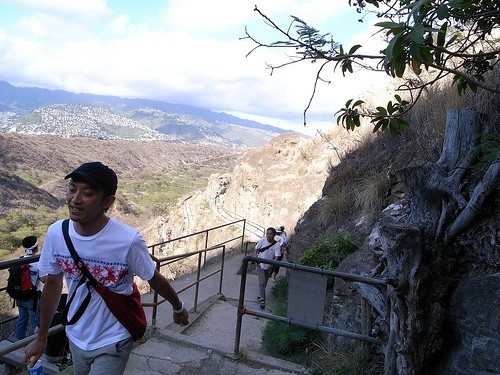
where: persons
[279,226,287,258]
[21,162,189,375]
[254,227,281,308]
[270,235,283,279]
[7,235,48,340]
[273,229,284,261]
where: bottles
[27,356,45,375]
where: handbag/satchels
[94,281,147,341]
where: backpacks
[6,256,39,300]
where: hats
[274,226,282,232]
[64,162,118,195]
[280,226,285,229]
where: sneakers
[260,300,265,309]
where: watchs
[173,302,185,314]
